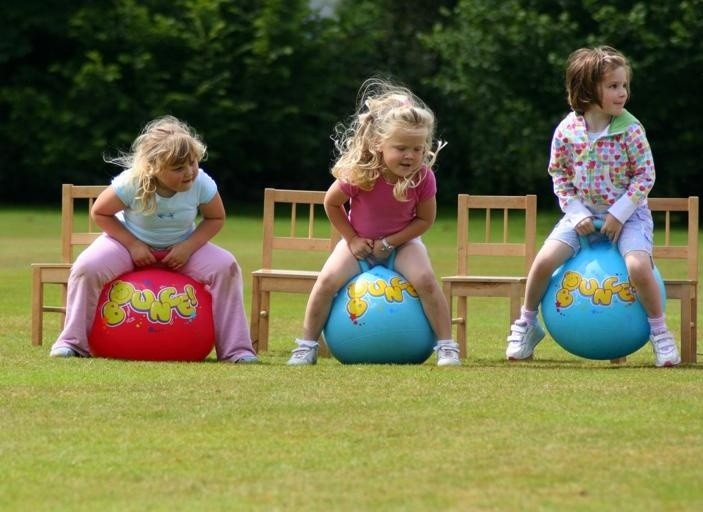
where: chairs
[441,193,537,360]
[611,196,699,365]
[30,183,111,346]
[250,188,342,360]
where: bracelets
[382,238,394,252]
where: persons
[285,78,462,370]
[47,116,262,365]
[502,45,683,367]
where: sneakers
[239,356,257,363]
[50,347,75,357]
[649,327,682,367]
[506,316,545,360]
[288,344,317,365]
[432,342,460,366]
